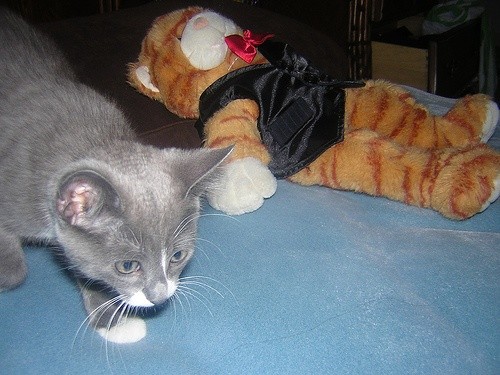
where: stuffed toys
[124,6,500,222]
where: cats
[0,0,236,343]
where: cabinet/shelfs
[369,19,486,108]
